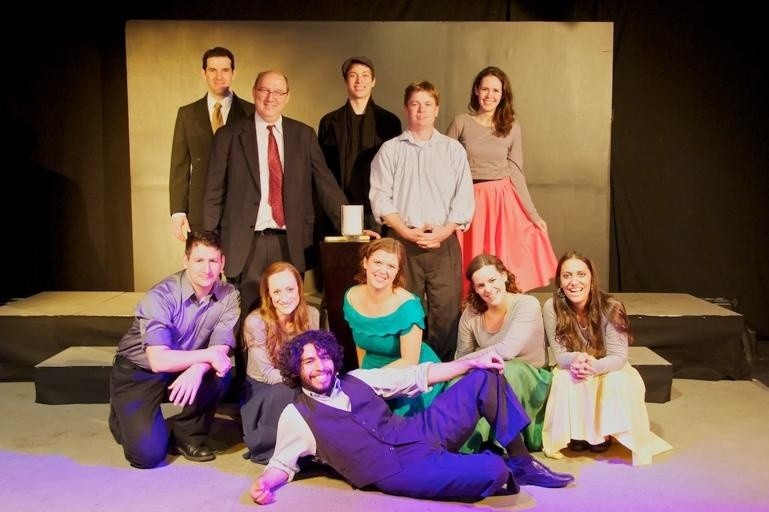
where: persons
[247,327,574,506]
[197,71,382,433]
[168,47,254,242]
[237,260,327,466]
[444,64,561,302]
[538,248,673,469]
[437,254,551,453]
[339,235,448,419]
[365,78,478,362]
[105,228,242,470]
[312,54,402,294]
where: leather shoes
[508,457,575,490]
[169,429,216,462]
[567,435,613,453]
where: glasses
[254,86,289,97]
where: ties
[267,125,284,227]
[210,104,224,136]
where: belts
[252,228,287,238]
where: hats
[342,55,375,74]
